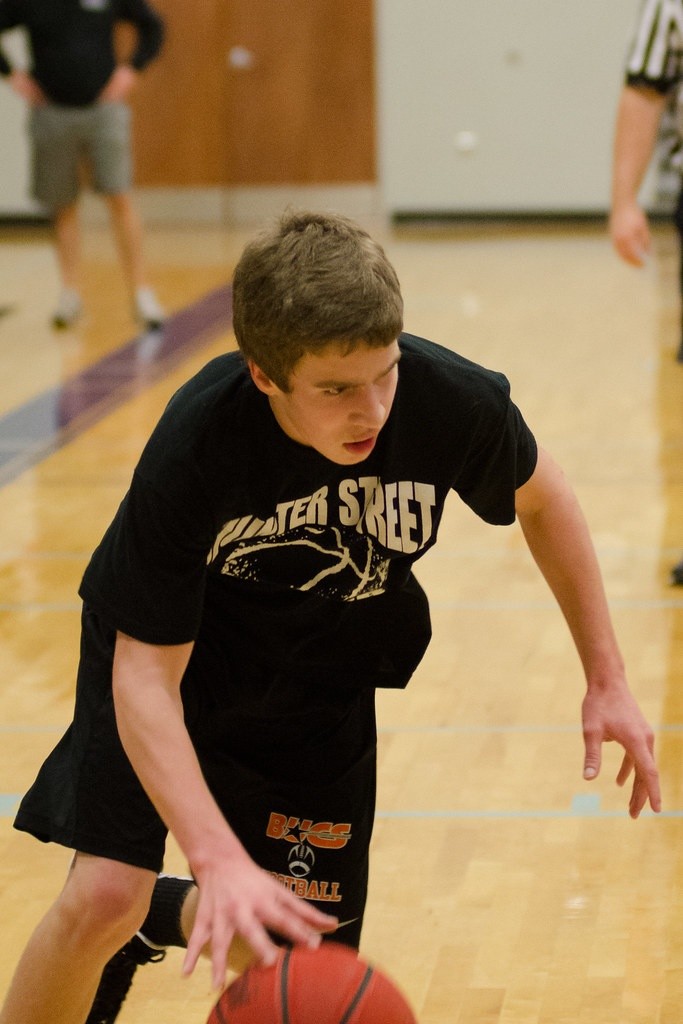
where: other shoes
[132,290,164,324]
[51,294,79,328]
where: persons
[0,0,164,328]
[0,212,662,1024]
[610,0,682,590]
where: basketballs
[206,939,417,1024]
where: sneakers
[83,932,168,1024]
